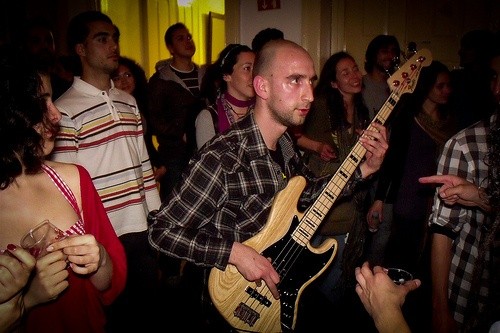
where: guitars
[206,46,434,333]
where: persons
[148,38,388,332]
[111,56,147,94]
[0,243,36,333]
[48,11,162,240]
[356,34,400,124]
[146,22,205,202]
[286,52,369,279]
[194,44,255,148]
[0,47,126,332]
[356,101,500,332]
[367,60,460,276]
[426,50,500,332]
